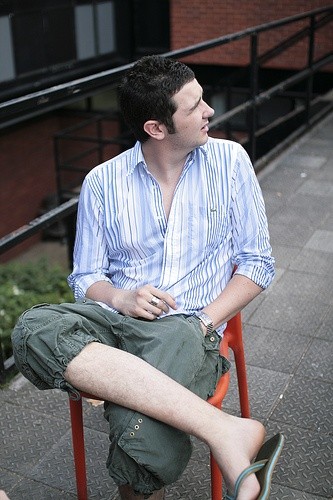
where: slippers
[221,432,285,500]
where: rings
[151,297,159,307]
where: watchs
[198,311,213,338]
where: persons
[11,55,288,499]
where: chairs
[66,266,249,499]
